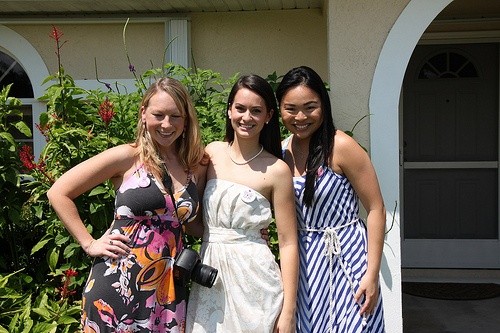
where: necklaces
[228,141,263,165]
[296,149,305,159]
[276,66,386,333]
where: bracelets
[88,239,96,255]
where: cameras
[173,246,218,288]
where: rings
[110,240,113,245]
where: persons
[47,77,209,333]
[184,74,300,333]
